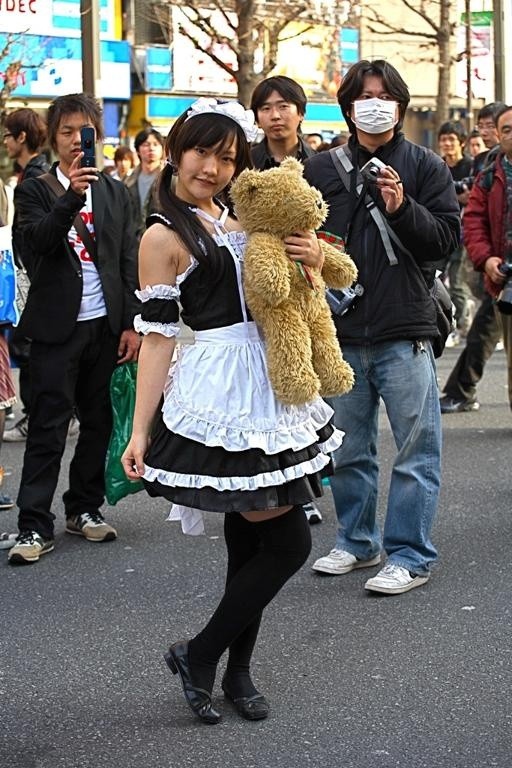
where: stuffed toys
[229,155,360,403]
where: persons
[6,94,128,567]
[120,96,346,727]
[0,336,17,454]
[235,75,333,527]
[293,58,457,597]
[158,133,174,162]
[2,107,81,442]
[438,104,511,413]
[305,132,327,151]
[122,128,169,226]
[462,105,512,424]
[0,174,17,420]
[108,145,141,183]
[468,129,486,156]
[438,121,474,349]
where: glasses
[4,134,12,139]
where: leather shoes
[221,672,269,720]
[164,641,221,723]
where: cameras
[449,176,477,194]
[493,257,512,312]
[316,282,356,317]
[359,158,386,186]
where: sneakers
[64,510,118,542]
[441,395,480,413]
[3,415,29,442]
[0,530,19,549]
[364,564,431,594]
[0,491,14,508]
[312,548,381,575]
[303,502,323,523]
[7,530,56,561]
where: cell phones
[79,126,97,181]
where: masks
[351,98,400,133]
